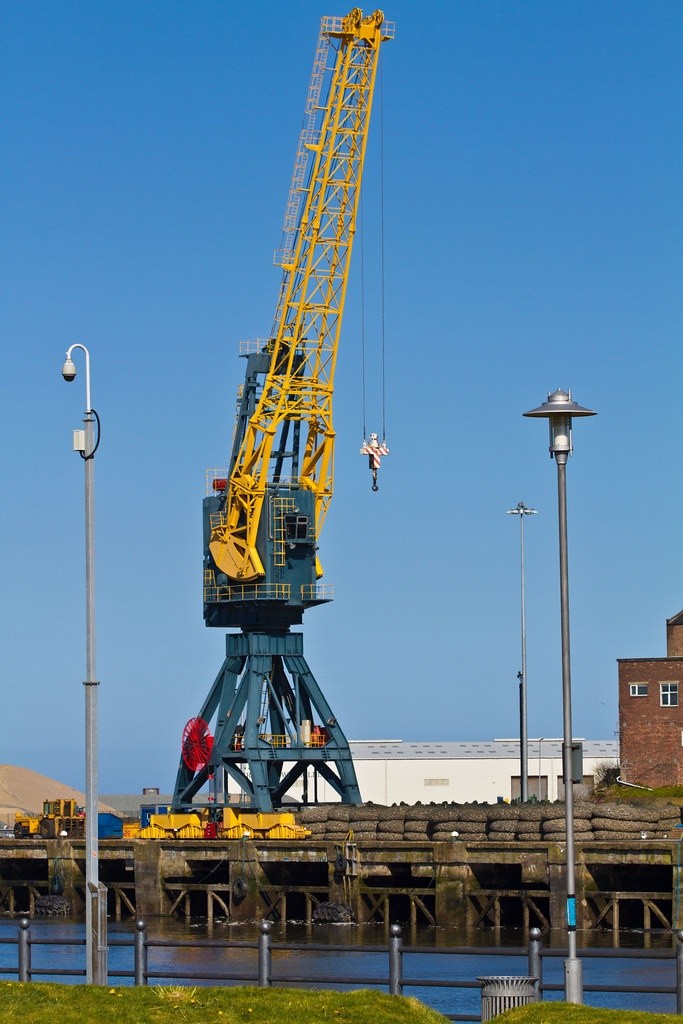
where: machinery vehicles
[13,798,85,840]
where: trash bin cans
[475,975,540,1023]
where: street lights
[505,499,538,802]
[520,385,601,1010]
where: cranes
[167,4,403,814]
